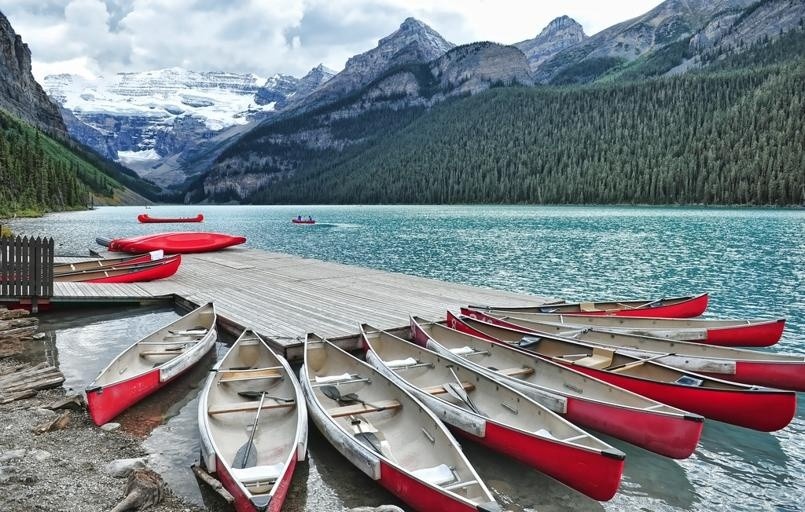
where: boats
[299,331,504,512]
[292,218,316,224]
[445,291,805,432]
[85,300,218,427]
[1,231,249,284]
[197,328,309,512]
[408,313,706,461]
[359,320,627,503]
[138,213,204,224]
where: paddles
[443,364,489,418]
[607,352,675,370]
[231,390,295,470]
[320,385,388,460]
